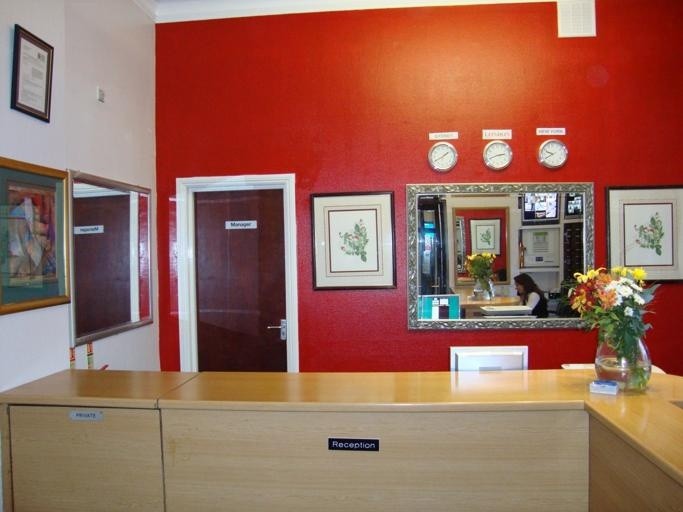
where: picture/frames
[310,190,396,291]
[11,23,54,123]
[468,218,503,257]
[604,185,683,284]
[0,156,71,315]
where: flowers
[466,251,495,298]
[559,266,655,386]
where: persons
[512,273,549,319]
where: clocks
[427,142,458,173]
[538,139,569,169]
[482,140,512,171]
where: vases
[473,277,495,300]
[595,333,651,390]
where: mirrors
[68,168,152,348]
[406,182,595,330]
